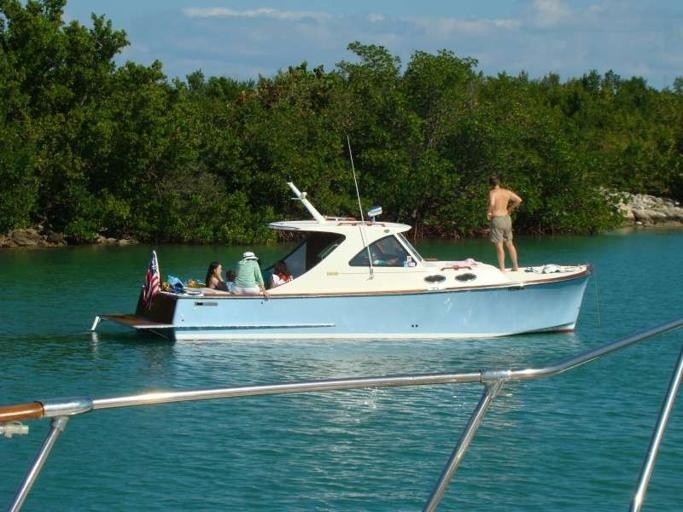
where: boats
[94,132,595,345]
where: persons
[265,259,293,290]
[205,261,225,289]
[485,174,522,272]
[223,270,235,291]
[230,251,270,300]
[368,252,400,266]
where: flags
[139,250,159,312]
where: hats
[241,250,259,260]
